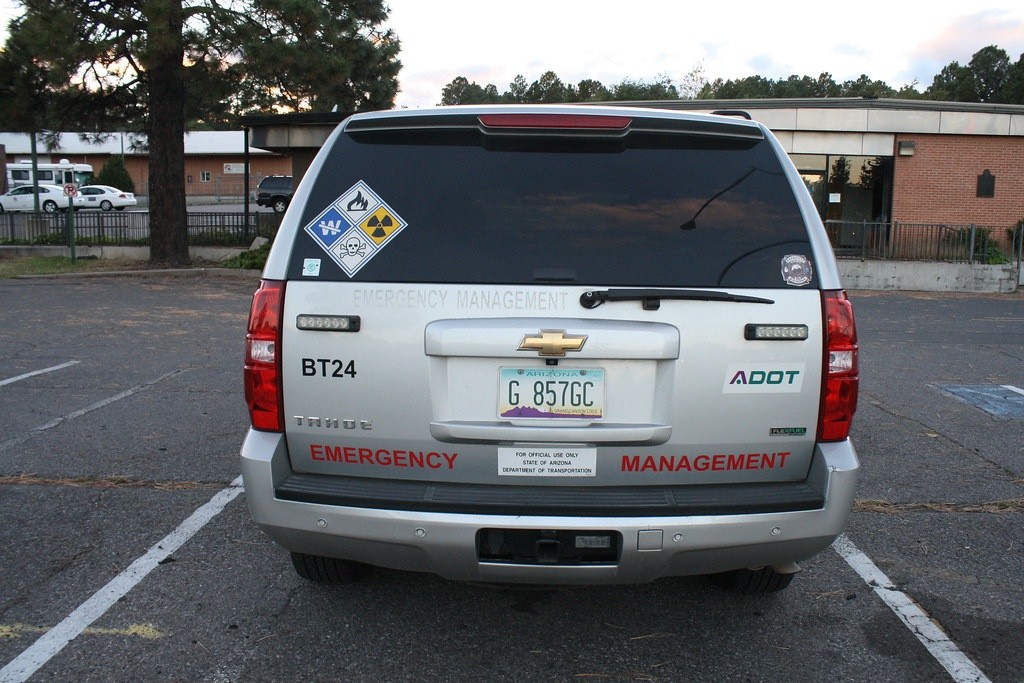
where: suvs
[255,174,294,213]
[238,107,859,596]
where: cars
[73,185,137,212]
[0,184,84,213]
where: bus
[6,159,94,191]
